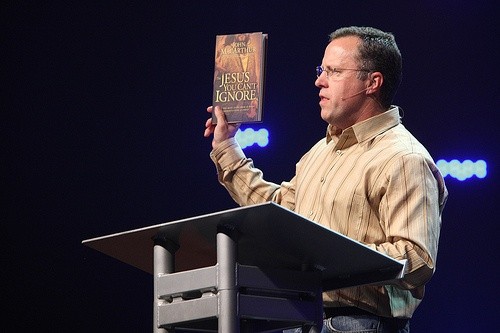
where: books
[208,32,266,124]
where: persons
[204,26,449,333]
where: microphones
[340,85,371,100]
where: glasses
[314,66,372,77]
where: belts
[324,307,373,319]
